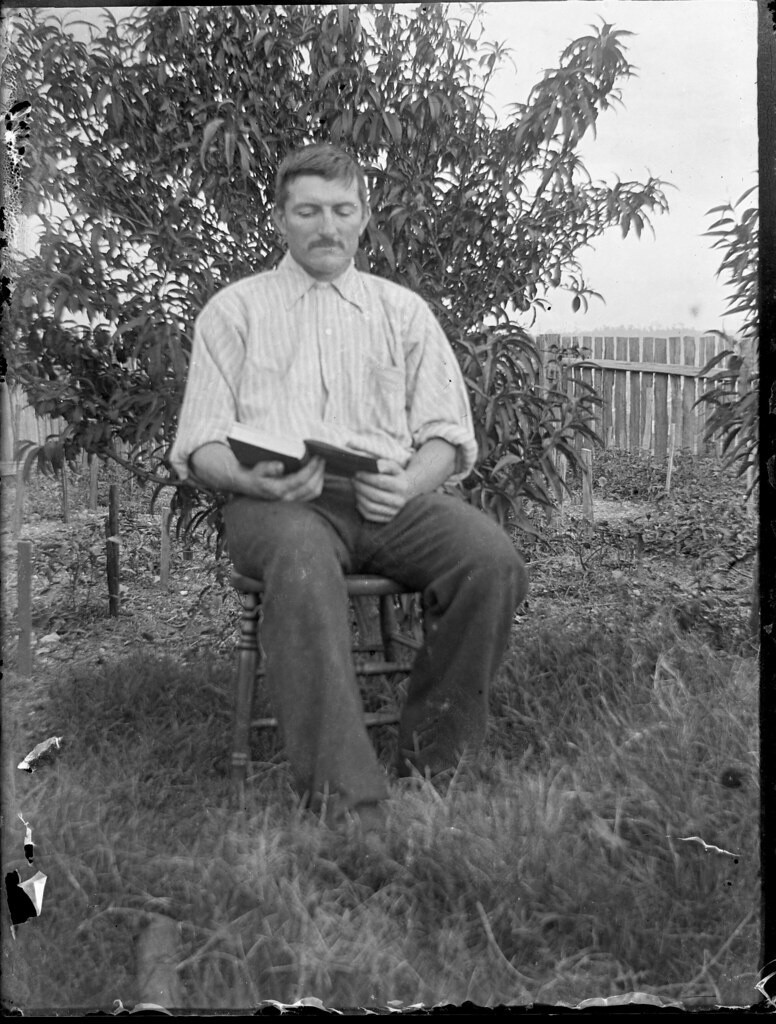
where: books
[228,421,381,473]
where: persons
[170,143,526,834]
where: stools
[228,562,416,778]
